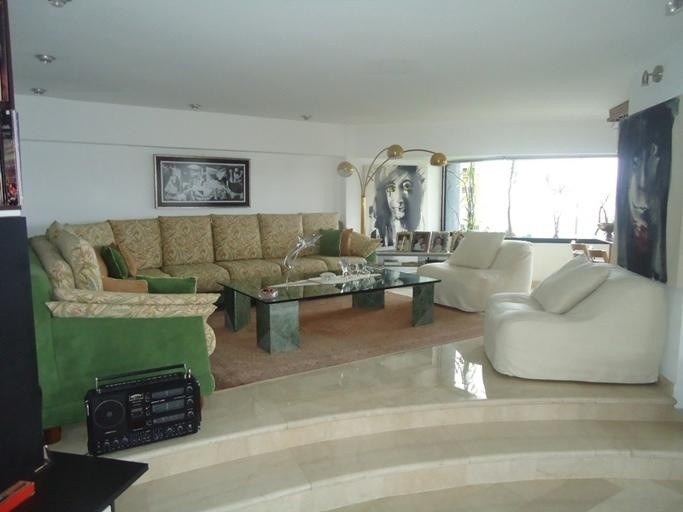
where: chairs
[570,238,610,264]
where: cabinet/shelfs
[374,246,453,267]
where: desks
[0,448,151,512]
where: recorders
[85,363,204,457]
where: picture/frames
[149,152,250,211]
[448,229,466,253]
[394,230,412,253]
[426,230,451,254]
[410,230,431,252]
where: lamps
[640,63,665,90]
[337,142,450,238]
[662,0,682,16]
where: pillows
[441,229,508,271]
[100,241,131,281]
[112,239,138,278]
[99,274,149,294]
[45,219,104,293]
[527,252,609,316]
[346,228,381,258]
[317,225,344,258]
[326,226,353,257]
[133,271,198,295]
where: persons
[372,165,423,247]
[614,104,671,281]
[412,235,426,250]
[431,234,443,251]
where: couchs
[476,256,668,386]
[52,208,376,311]
[413,237,535,316]
[26,220,217,448]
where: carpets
[188,290,487,399]
